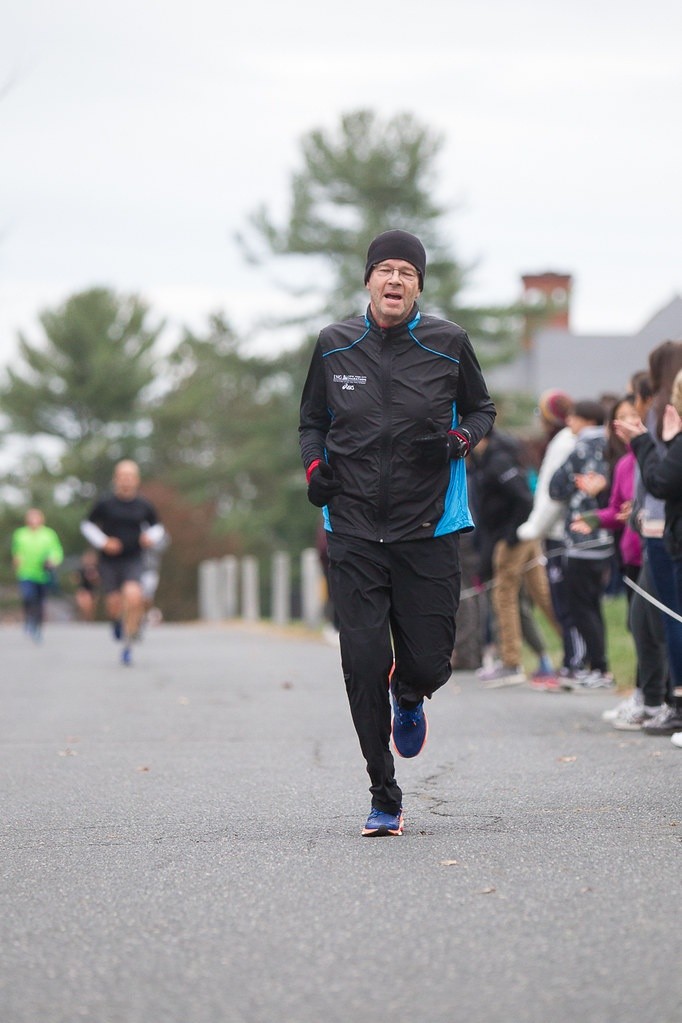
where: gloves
[305,459,340,507]
[407,417,469,469]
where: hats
[541,390,573,428]
[363,231,426,291]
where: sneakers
[362,805,407,837]
[670,732,682,746]
[615,703,671,732]
[527,672,554,689]
[606,696,636,722]
[388,658,427,758]
[567,670,618,693]
[480,664,521,687]
[643,706,682,735]
[559,668,588,687]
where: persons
[298,229,494,836]
[9,459,168,669]
[317,341,681,748]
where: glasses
[372,263,420,281]
